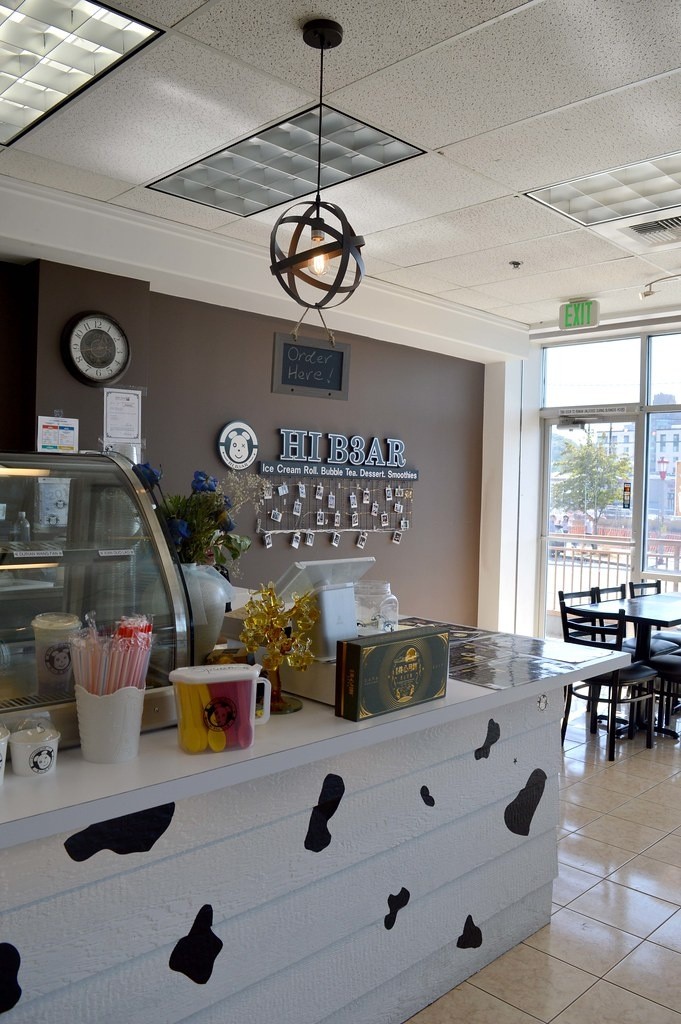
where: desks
[569,591,681,732]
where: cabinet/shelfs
[0,448,195,761]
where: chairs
[558,578,681,761]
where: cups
[74,682,146,764]
[0,729,10,788]
[31,613,82,687]
[9,730,60,776]
[170,665,271,756]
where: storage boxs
[334,626,450,721]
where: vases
[178,563,226,666]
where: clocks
[57,310,132,387]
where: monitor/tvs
[273,556,376,604]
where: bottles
[354,579,399,634]
[13,512,30,542]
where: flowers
[132,461,274,565]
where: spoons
[173,680,253,754]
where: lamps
[269,20,366,310]
